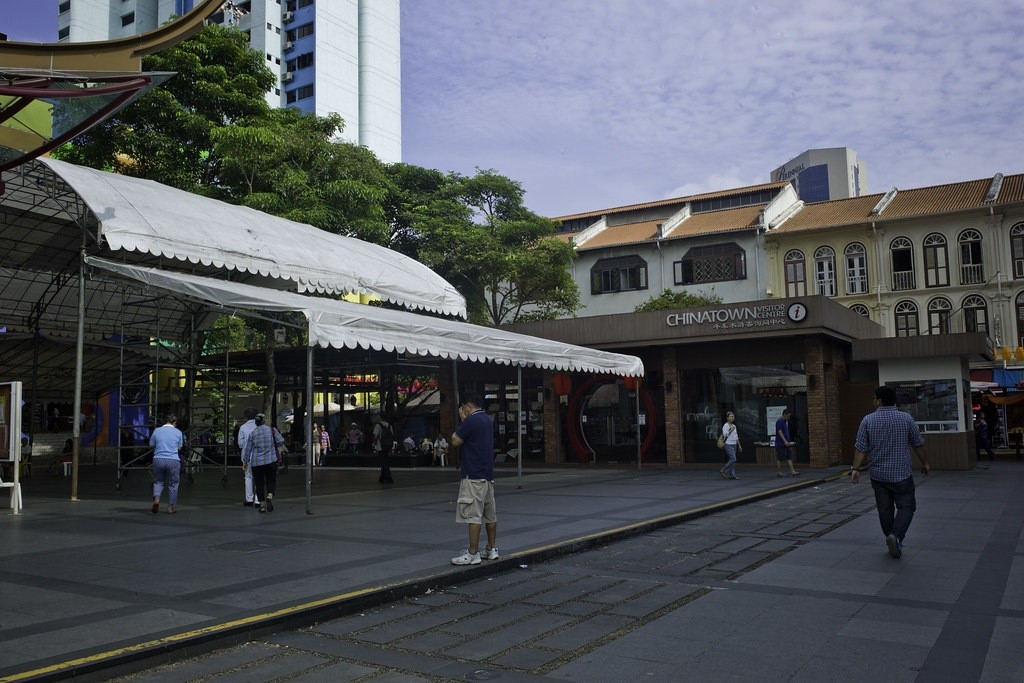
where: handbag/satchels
[272,427,289,467]
[443,447,450,456]
[717,435,725,450]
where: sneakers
[450,549,482,565]
[477,545,499,560]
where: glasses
[871,395,877,400]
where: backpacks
[376,422,395,453]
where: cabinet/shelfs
[991,408,1009,449]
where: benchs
[1008,432,1024,456]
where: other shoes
[789,471,802,475]
[776,473,791,477]
[151,501,160,514]
[168,507,176,514]
[729,476,739,480]
[255,504,260,508]
[886,533,903,559]
[720,471,728,479]
[244,500,253,507]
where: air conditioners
[282,42,293,53]
[281,71,294,82]
[282,11,294,23]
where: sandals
[258,507,266,513]
[266,496,274,513]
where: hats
[351,422,357,426]
[979,412,984,414]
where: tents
[86,258,643,486]
[718,365,808,395]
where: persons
[775,409,802,477]
[203,426,216,450]
[851,385,930,558]
[233,424,241,455]
[403,432,446,466]
[238,408,261,507]
[21,437,31,459]
[372,411,394,489]
[346,422,363,454]
[974,412,1000,460]
[149,413,183,514]
[62,439,74,462]
[720,411,743,480]
[118,424,135,477]
[277,442,289,457]
[47,398,95,433]
[309,422,332,467]
[145,410,188,458]
[451,391,500,565]
[243,414,285,513]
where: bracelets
[851,466,860,471]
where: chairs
[441,443,449,466]
[184,448,204,473]
[9,452,33,479]
[0,478,23,509]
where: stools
[61,461,72,476]
